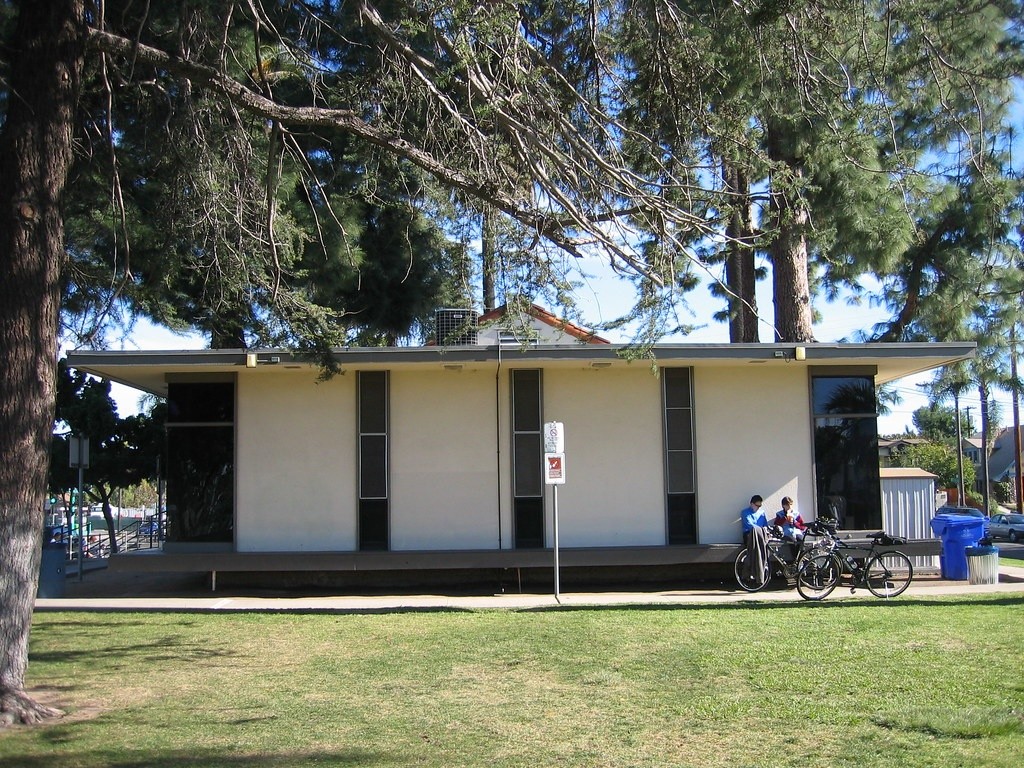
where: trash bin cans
[964,537,1000,586]
[930,506,990,581]
[37,543,69,599]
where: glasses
[754,503,762,507]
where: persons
[742,494,768,548]
[774,496,807,565]
[49,532,104,559]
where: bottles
[845,553,858,569]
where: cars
[988,513,1024,543]
[935,507,991,538]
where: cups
[786,513,793,524]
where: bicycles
[734,525,914,601]
